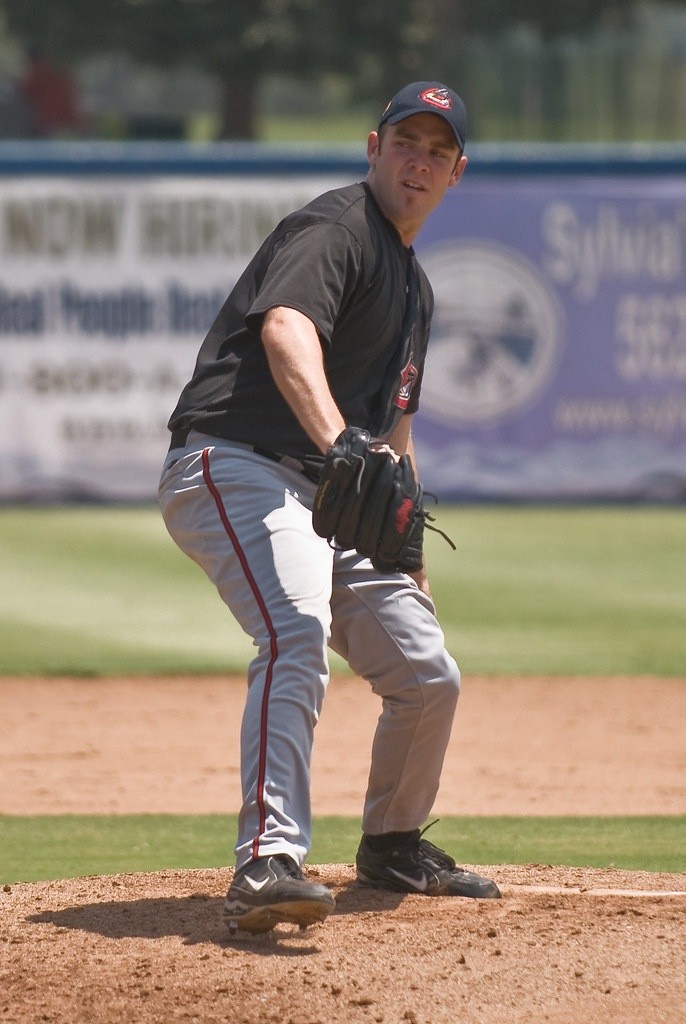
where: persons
[159,82,503,930]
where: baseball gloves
[306,425,431,573]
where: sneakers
[356,819,501,898]
[222,853,336,935]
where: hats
[378,81,468,154]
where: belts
[168,430,322,485]
